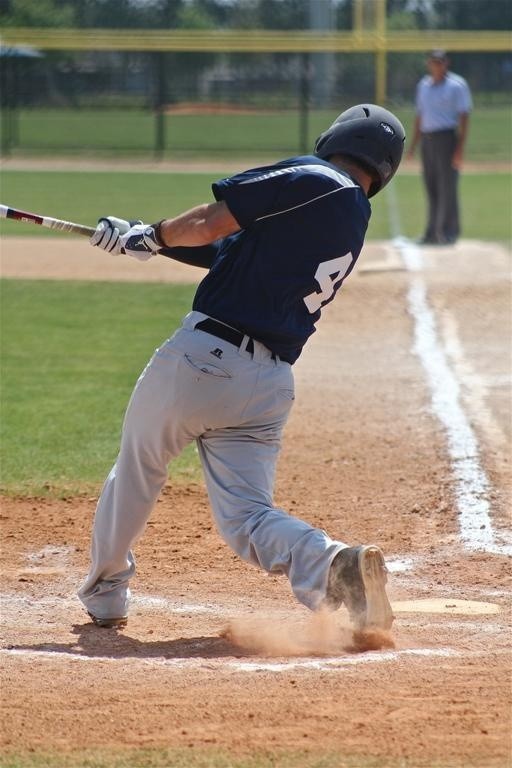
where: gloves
[90,217,144,255]
[123,219,169,262]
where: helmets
[314,103,405,198]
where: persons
[403,51,475,247]
[76,103,408,641]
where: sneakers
[333,544,392,640]
[87,609,128,627]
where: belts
[193,317,283,362]
